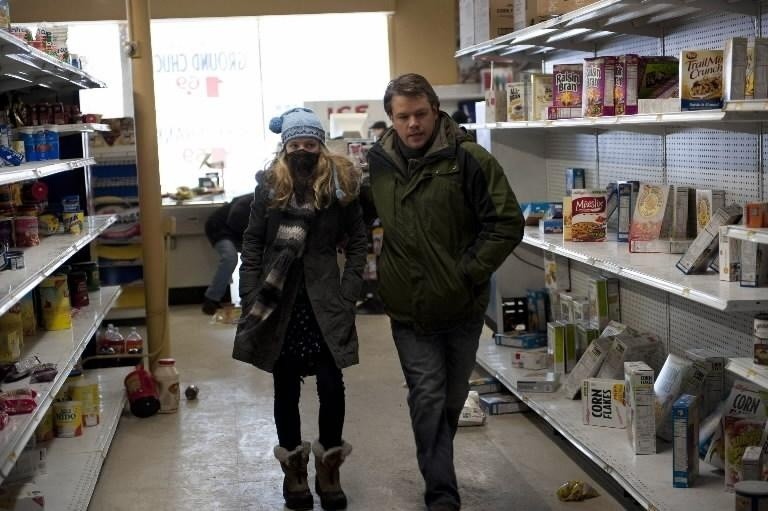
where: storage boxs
[460,0,600,50]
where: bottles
[98,323,144,356]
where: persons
[231,108,370,511]
[202,191,255,315]
[369,120,388,138]
[367,73,526,511]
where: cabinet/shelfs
[456,0,767,511]
[0,28,140,511]
[158,193,233,309]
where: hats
[271,107,325,145]
[369,121,386,129]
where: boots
[312,436,351,511]
[274,441,314,508]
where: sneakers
[201,298,223,317]
